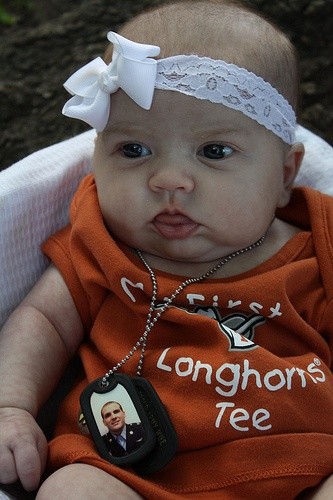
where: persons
[1,0,333,500]
[100,401,147,458]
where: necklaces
[79,229,267,473]
